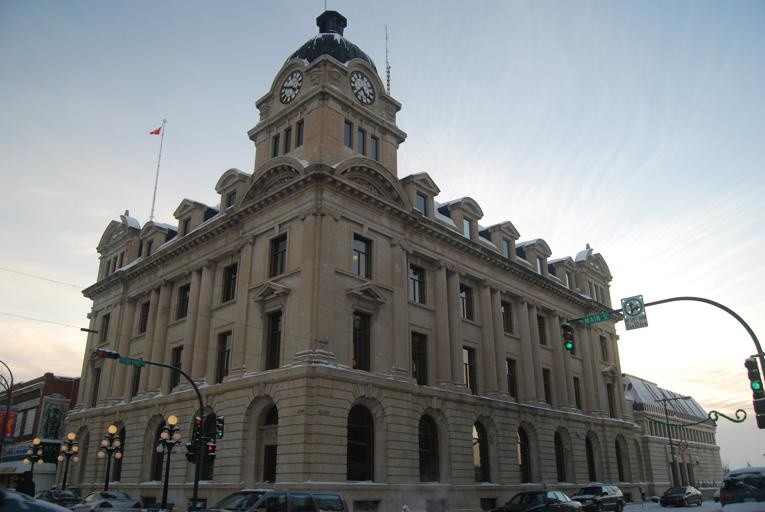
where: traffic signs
[583,312,612,326]
[118,354,146,369]
[620,294,649,332]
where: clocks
[279,69,303,105]
[349,70,375,105]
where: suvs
[204,489,351,511]
[719,466,765,511]
[571,484,627,512]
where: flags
[149,118,168,138]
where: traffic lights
[215,415,225,437]
[192,414,203,437]
[560,321,574,351]
[207,441,217,460]
[752,388,765,430]
[94,348,119,360]
[744,356,763,392]
[185,441,193,460]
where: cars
[492,487,581,512]
[713,487,722,502]
[0,489,143,512]
[661,484,703,507]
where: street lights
[55,431,80,487]
[22,436,45,473]
[96,424,122,490]
[153,413,182,507]
[654,396,692,485]
